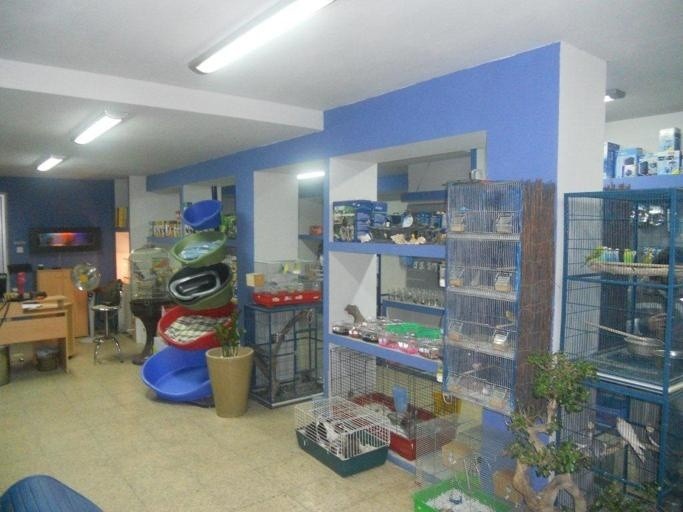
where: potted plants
[204,310,255,417]
[499,346,603,512]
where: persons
[624,246,683,482]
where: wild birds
[615,414,649,465]
[580,245,609,269]
[622,247,636,273]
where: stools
[92,303,124,364]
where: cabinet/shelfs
[322,175,476,476]
[440,179,557,411]
[143,184,249,402]
[35,267,89,338]
[545,185,683,507]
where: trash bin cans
[36,348,58,371]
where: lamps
[35,153,65,171]
[187,0,336,76]
[71,109,128,145]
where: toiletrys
[145,213,240,239]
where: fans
[71,260,105,345]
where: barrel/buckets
[181,198,224,230]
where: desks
[0,295,74,373]
